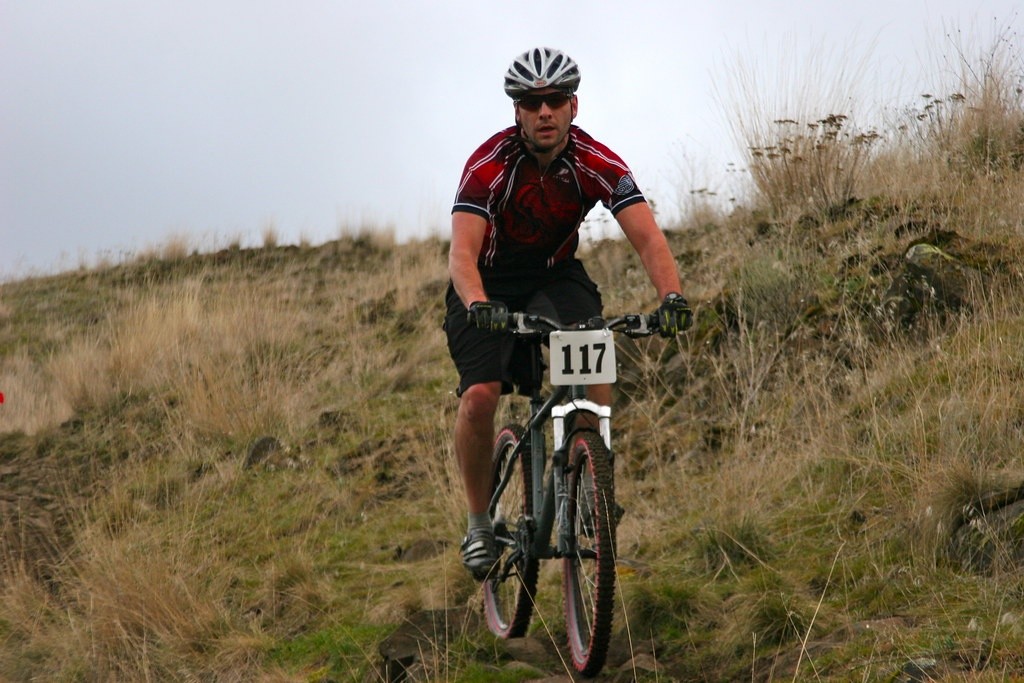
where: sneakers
[460,529,499,573]
[579,494,624,538]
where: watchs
[664,291,689,304]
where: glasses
[515,91,576,112]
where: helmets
[504,47,582,98]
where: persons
[441,45,691,576]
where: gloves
[651,292,692,338]
[467,299,508,333]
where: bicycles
[464,306,702,679]
